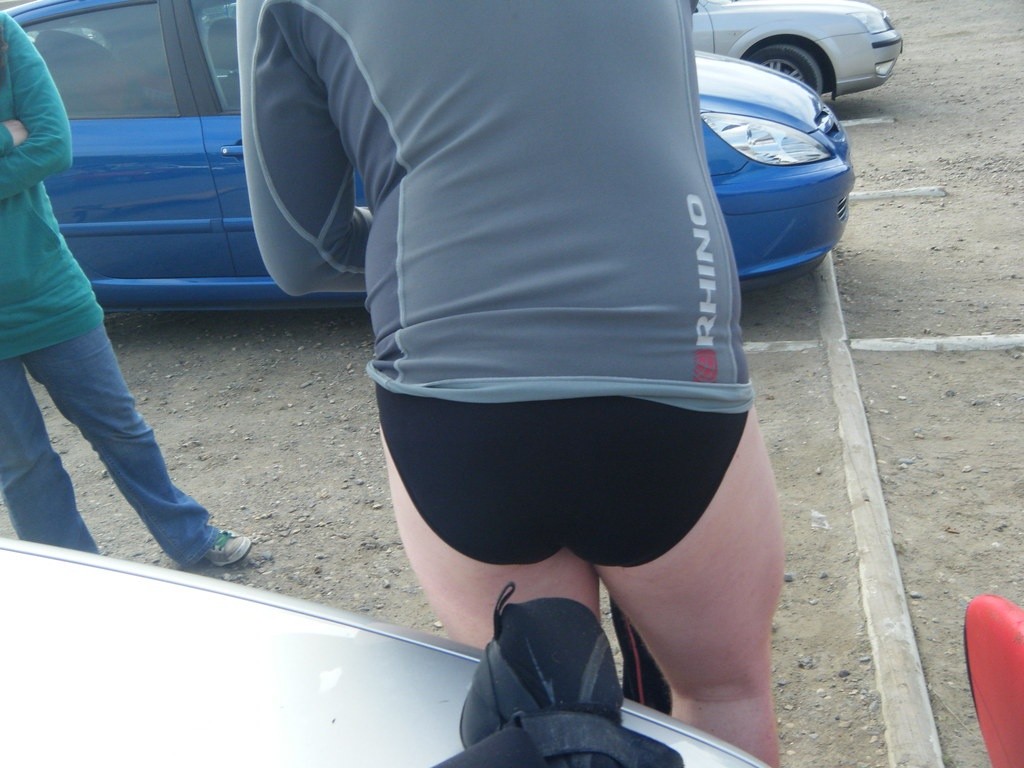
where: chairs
[32,18,241,117]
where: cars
[689,0,904,100]
[0,538,1024,768]
[1,1,854,312]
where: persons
[235,0,785,768]
[0,15,250,564]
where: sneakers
[203,529,252,567]
